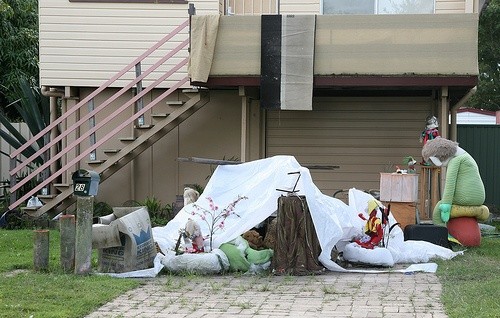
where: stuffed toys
[422,136,486,226]
[183,218,210,253]
[353,208,383,248]
[244,231,264,250]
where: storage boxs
[379,170,420,205]
[91,203,158,276]
[379,199,417,230]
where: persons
[420,115,440,167]
